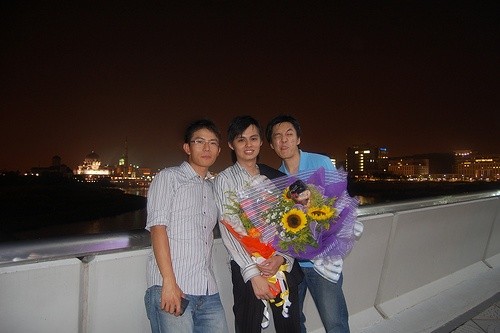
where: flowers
[220,165,361,301]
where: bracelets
[275,254,287,265]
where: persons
[266,115,350,333]
[144,118,228,333]
[214,115,307,333]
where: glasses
[189,139,220,147]
